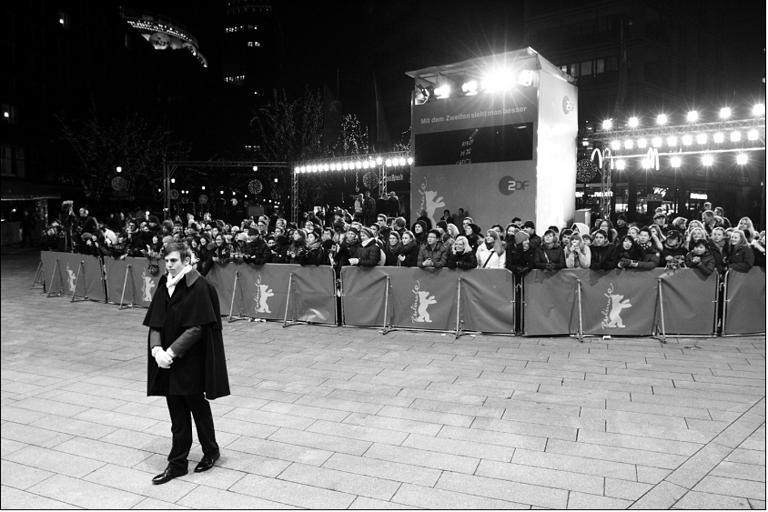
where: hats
[348,227,358,235]
[515,231,531,245]
[360,227,374,238]
[666,230,680,239]
[416,220,428,233]
[436,221,449,232]
[395,217,407,227]
[467,223,482,233]
[247,228,261,236]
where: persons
[142,241,232,485]
[43,193,765,337]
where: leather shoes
[152,461,188,484]
[194,451,220,472]
